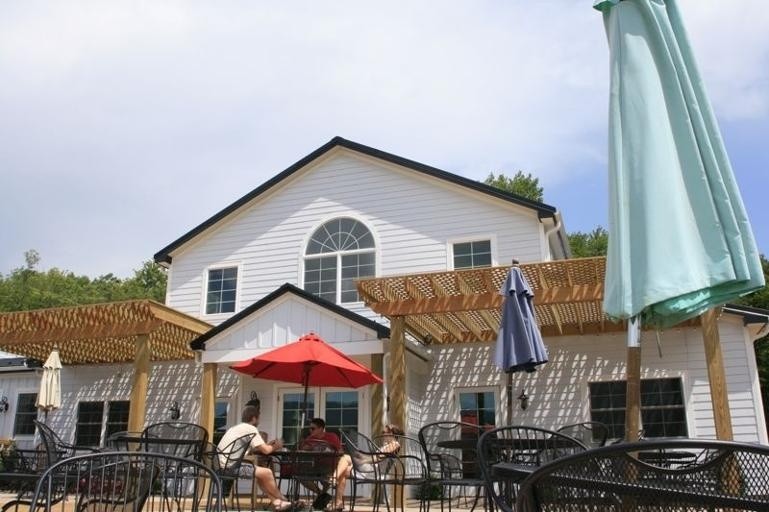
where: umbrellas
[33,348,64,426]
[590,0,767,485]
[227,328,384,503]
[491,255,551,463]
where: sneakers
[310,491,333,511]
[322,501,345,512]
[321,474,339,488]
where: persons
[298,418,344,494]
[216,405,304,511]
[322,424,406,511]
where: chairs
[205,440,258,511]
[0,419,209,512]
[289,440,339,511]
[302,426,345,511]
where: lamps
[168,401,181,422]
[0,394,9,413]
[515,388,530,411]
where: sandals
[272,498,308,512]
